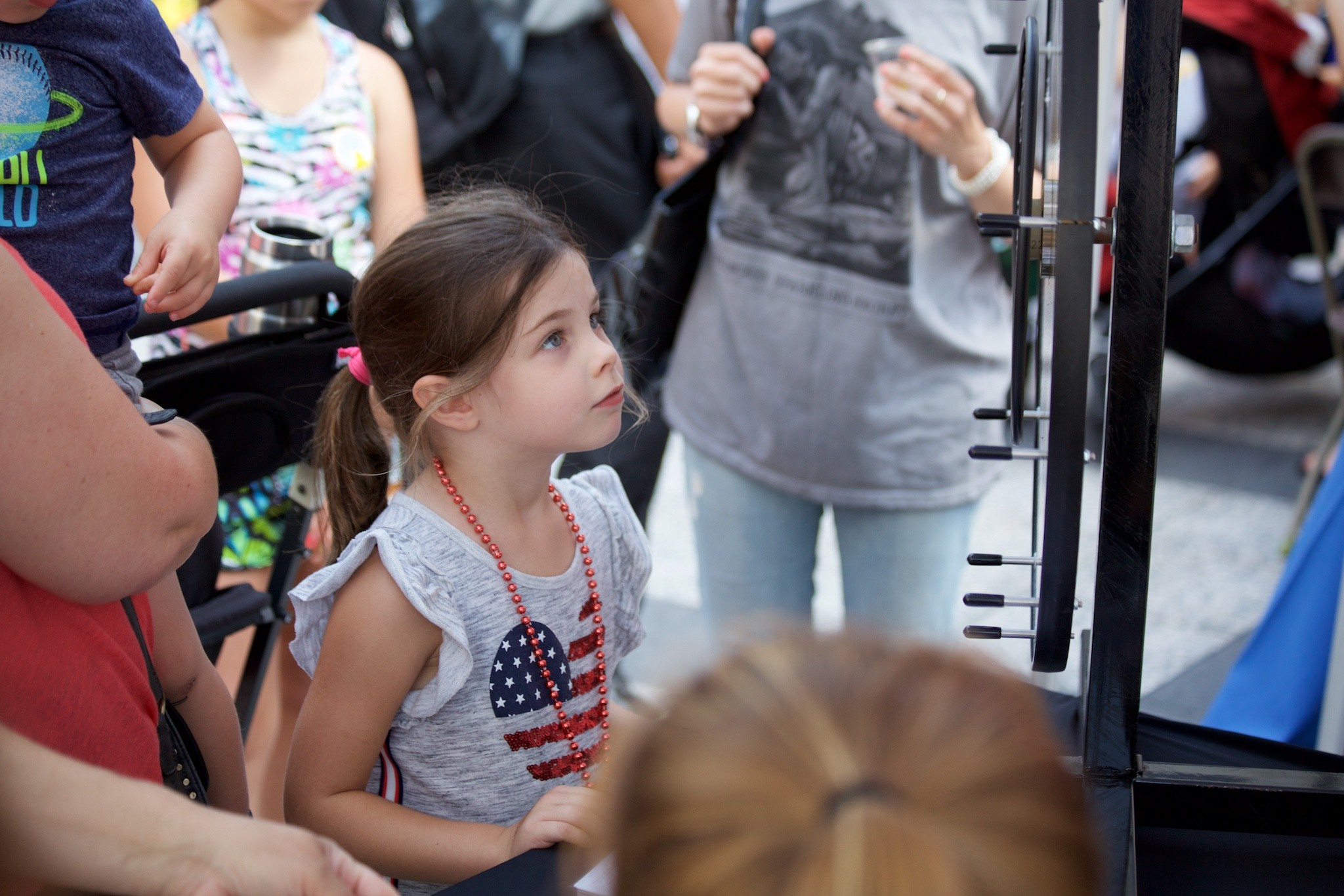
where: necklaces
[435,454,612,789]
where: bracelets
[949,128,1012,196]
[685,98,718,146]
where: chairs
[1093,0,1343,572]
[126,267,365,750]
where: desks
[427,676,1344,896]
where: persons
[0,0,1344,896]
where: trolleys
[66,259,411,834]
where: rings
[934,89,947,106]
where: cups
[226,214,336,344]
